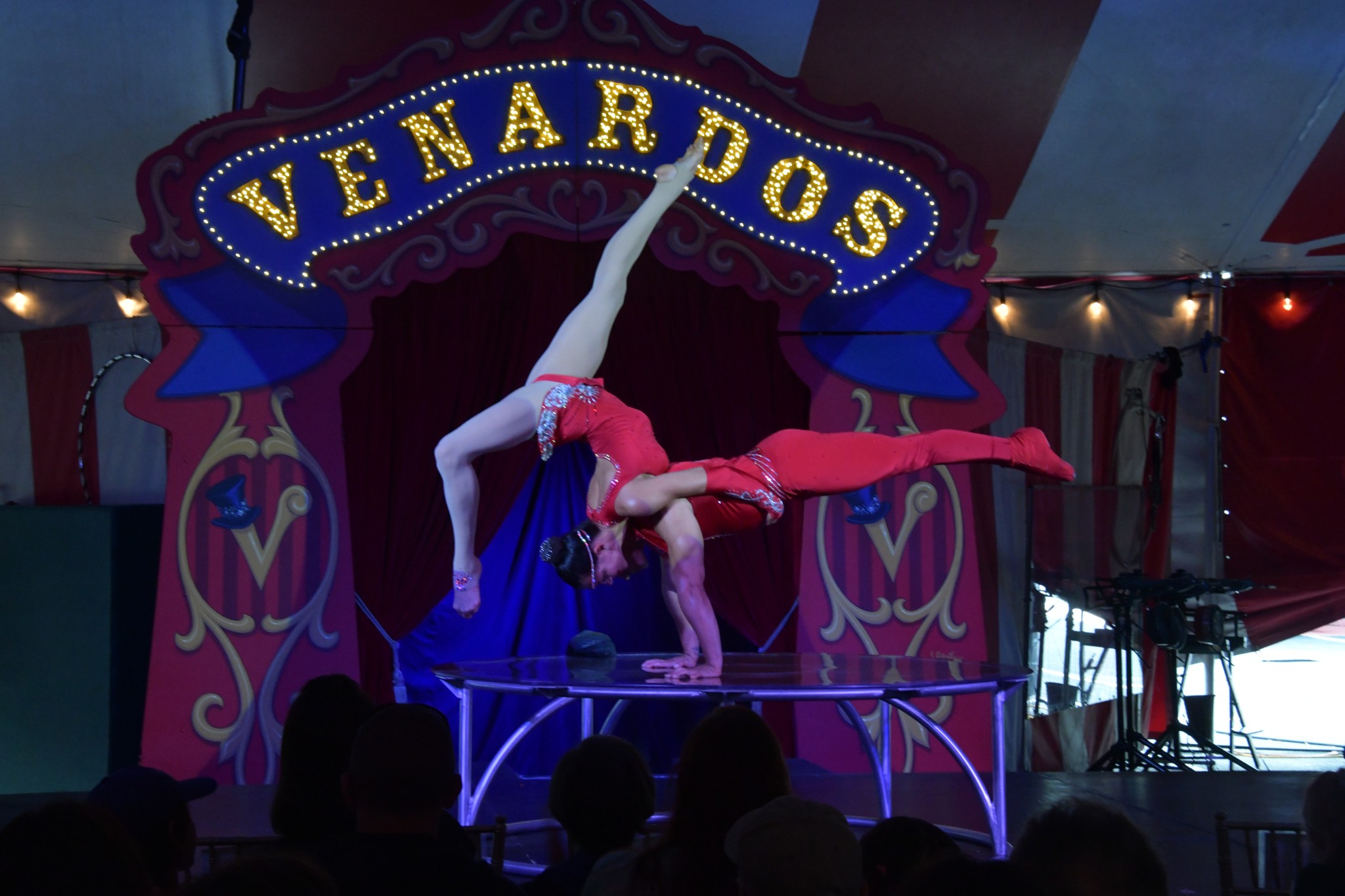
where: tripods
[1079,571,1264,773]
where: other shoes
[1006,427,1075,481]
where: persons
[434,134,785,622]
[621,426,1075,681]
[80,668,1345,896]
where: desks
[429,644,1028,861]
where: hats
[88,767,219,817]
